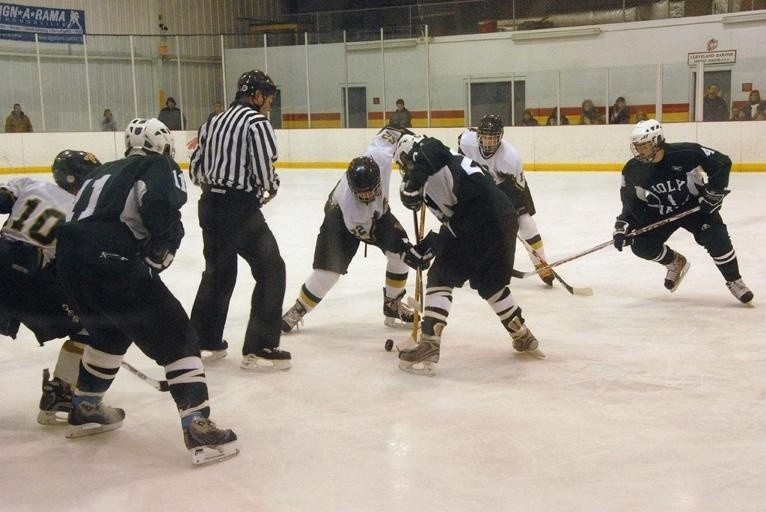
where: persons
[208,99,227,121]
[546,107,569,125]
[389,99,411,128]
[608,97,629,124]
[704,84,766,122]
[158,97,187,131]
[579,100,605,125]
[390,134,539,364]
[613,116,753,302]
[634,110,648,124]
[4,104,34,134]
[518,110,539,126]
[187,69,293,361]
[101,108,117,131]
[457,114,554,286]
[278,125,430,334]
[1,149,90,412]
[55,118,240,450]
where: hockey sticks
[394,199,427,350]
[510,204,701,278]
[408,211,424,313]
[120,360,169,393]
[515,235,594,296]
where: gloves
[404,230,446,270]
[373,223,412,253]
[690,183,731,216]
[134,246,176,280]
[400,172,421,211]
[613,217,636,251]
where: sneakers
[665,251,686,289]
[536,265,554,287]
[241,345,291,370]
[508,316,545,357]
[726,275,753,303]
[40,382,237,450]
[200,340,228,363]
[399,323,444,377]
[383,287,421,328]
[280,299,307,333]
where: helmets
[346,154,384,203]
[630,119,665,164]
[477,113,504,140]
[125,118,175,157]
[237,70,280,97]
[393,133,427,173]
[53,150,101,190]
